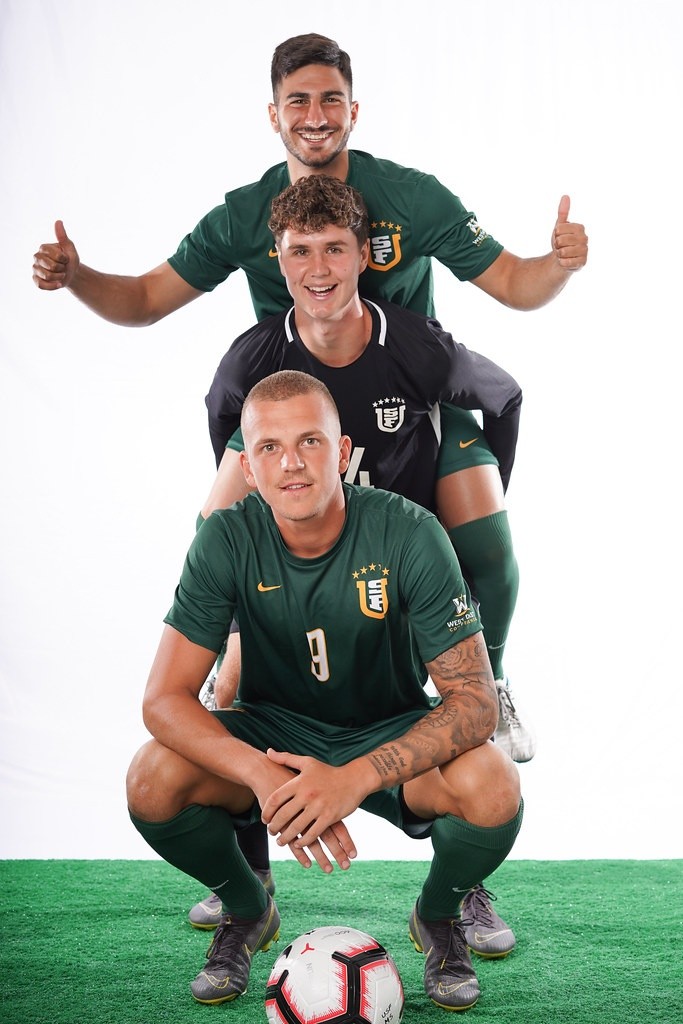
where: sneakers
[199,673,221,712]
[189,867,275,930]
[488,674,534,764]
[407,894,481,1012]
[190,891,283,1005]
[460,881,517,960]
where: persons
[185,173,526,960]
[31,33,589,762]
[122,369,524,1012]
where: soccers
[264,925,408,1024]
